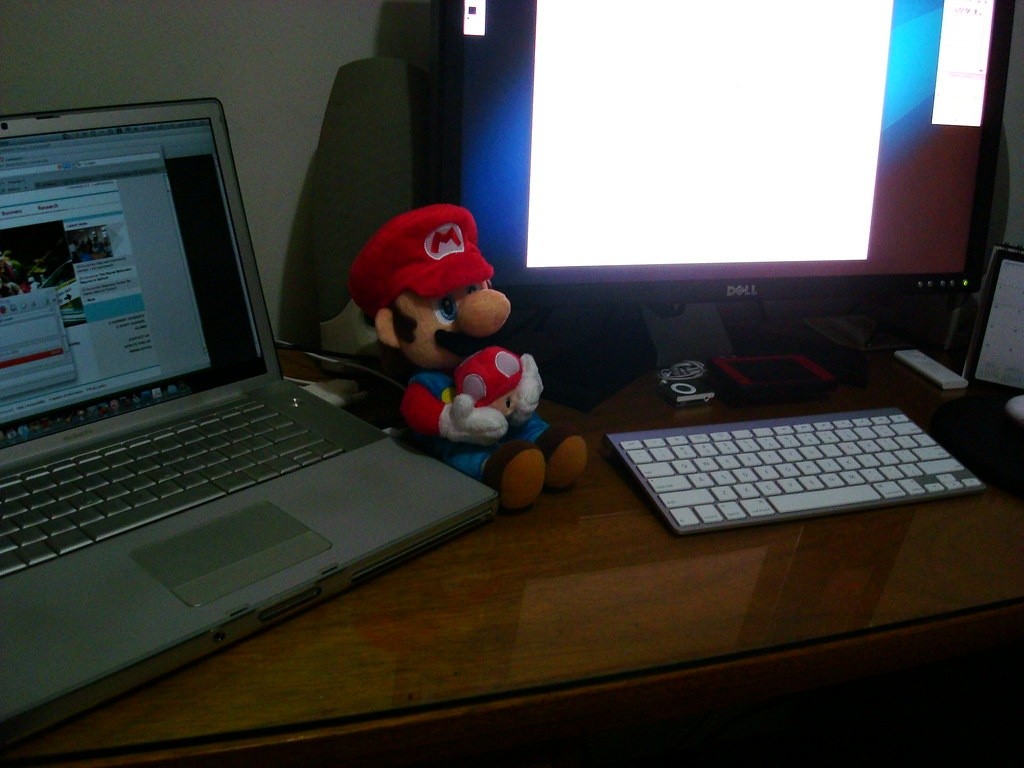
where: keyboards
[606,408,985,536]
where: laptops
[0,98,499,757]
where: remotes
[892,350,968,389]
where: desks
[0,351,1024,768]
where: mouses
[1006,395,1024,424]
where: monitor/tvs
[406,1,1016,408]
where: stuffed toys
[347,203,589,508]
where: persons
[0,251,25,298]
